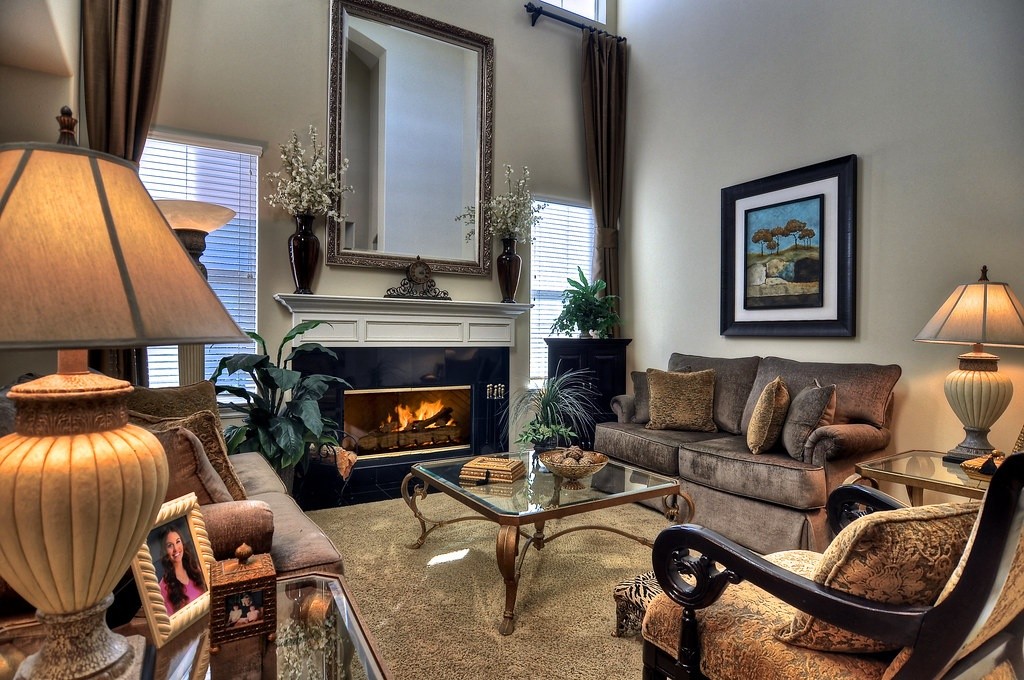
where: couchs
[590,350,902,552]
[0,372,345,575]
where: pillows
[126,408,248,503]
[646,367,983,653]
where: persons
[226,596,260,626]
[159,525,206,615]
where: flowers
[261,125,549,246]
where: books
[460,457,527,482]
[459,476,526,496]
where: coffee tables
[401,450,693,636]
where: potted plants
[210,319,358,494]
[499,266,629,463]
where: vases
[287,213,522,306]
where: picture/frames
[131,491,216,649]
[720,153,857,337]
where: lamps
[912,265,1024,459]
[0,105,255,680]
[154,199,235,233]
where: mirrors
[326,0,494,279]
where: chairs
[644,458,1024,680]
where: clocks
[382,255,452,299]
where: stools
[612,570,697,639]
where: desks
[0,572,393,680]
[855,450,989,507]
